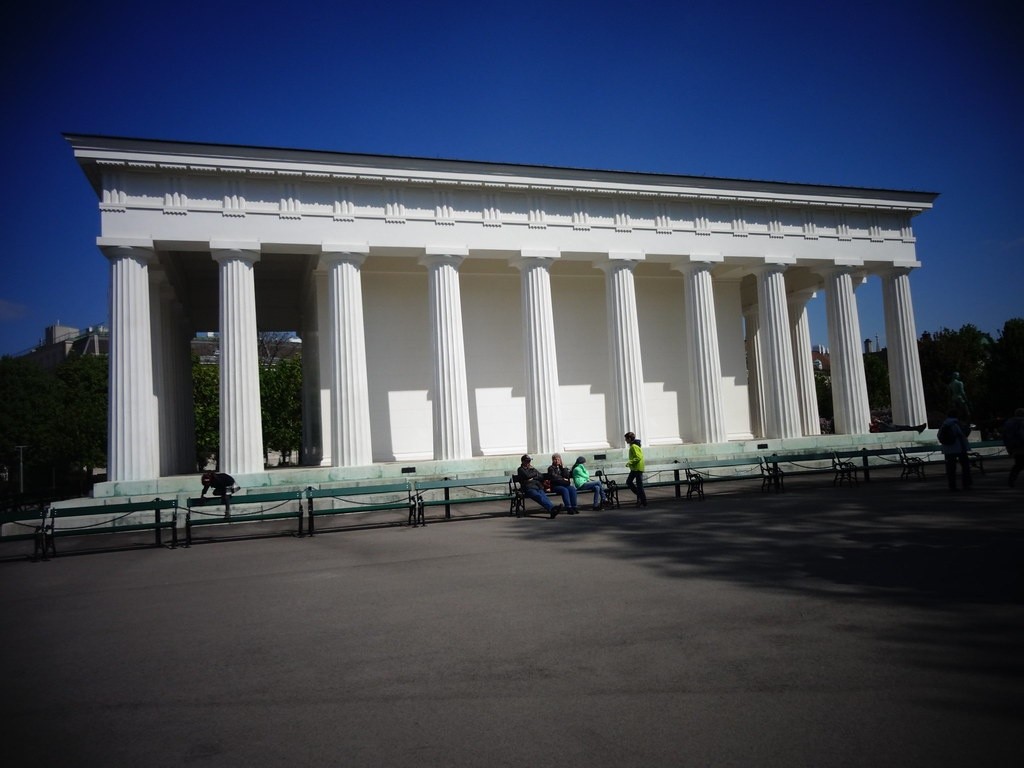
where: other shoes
[593,507,601,511]
[917,423,926,433]
[1008,482,1016,488]
[636,495,647,506]
[949,488,960,492]
[567,509,580,514]
[604,501,610,505]
[551,503,563,519]
[967,487,975,491]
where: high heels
[223,512,231,519]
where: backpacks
[941,425,955,443]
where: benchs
[33,499,177,565]
[414,476,516,528]
[603,463,701,508]
[763,452,850,489]
[306,481,417,538]
[507,468,619,518]
[184,489,304,548]
[684,458,778,496]
[836,439,1005,488]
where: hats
[952,372,960,376]
[521,454,533,460]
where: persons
[820,416,835,434]
[518,454,562,519]
[548,454,579,515]
[937,415,977,492]
[1002,408,1024,487]
[625,432,648,508]
[927,411,1002,441]
[871,419,926,433]
[942,371,976,423]
[201,472,241,498]
[572,456,608,511]
